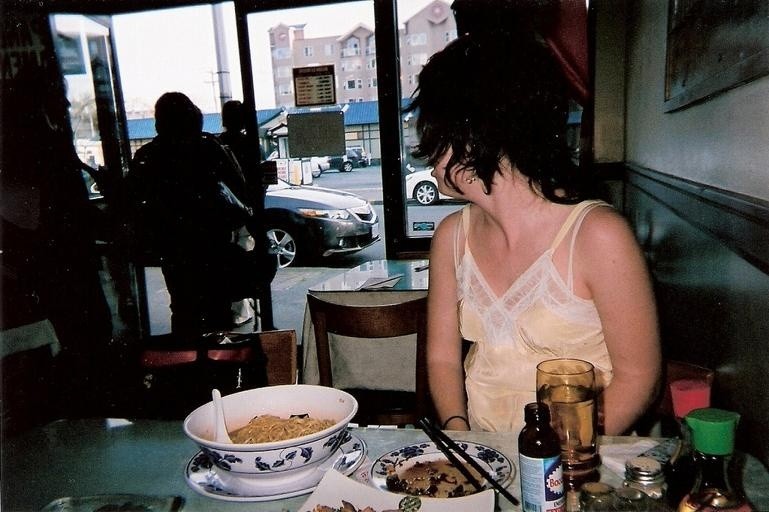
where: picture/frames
[660,0,769,115]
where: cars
[403,165,469,209]
[264,143,371,180]
[77,164,382,267]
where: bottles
[579,378,754,512]
[517,401,567,512]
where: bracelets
[441,416,471,431]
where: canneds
[563,454,601,512]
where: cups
[534,357,599,488]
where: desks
[306,258,429,392]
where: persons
[124,92,259,337]
[403,27,665,437]
[0,64,133,421]
[215,101,269,330]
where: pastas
[234,415,335,443]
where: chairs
[307,294,428,430]
[216,330,297,385]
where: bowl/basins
[181,380,359,484]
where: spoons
[211,388,235,445]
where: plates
[298,469,497,510]
[38,490,185,510]
[370,441,515,499]
[184,430,368,502]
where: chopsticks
[417,415,518,510]
[366,275,404,286]
[414,262,430,273]
